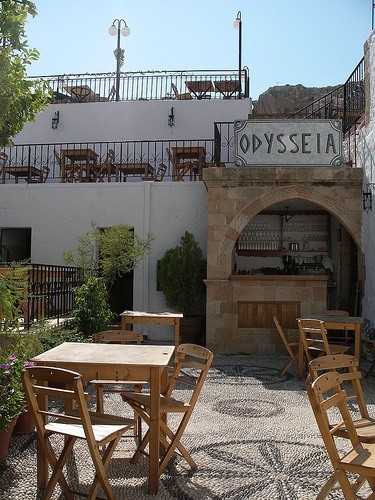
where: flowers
[0,346,34,431]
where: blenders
[282,255,292,273]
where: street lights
[108,19,130,101]
[233,11,242,98]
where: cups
[238,220,328,251]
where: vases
[0,395,37,461]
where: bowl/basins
[261,267,277,275]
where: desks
[296,316,364,381]
[2,166,41,183]
[116,163,155,181]
[64,85,97,103]
[172,147,207,181]
[119,310,183,369]
[30,342,176,495]
[62,149,99,182]
[185,81,242,100]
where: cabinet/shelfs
[235,210,331,257]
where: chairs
[0,148,225,184]
[171,83,193,100]
[20,330,213,500]
[272,310,375,500]
[97,85,116,102]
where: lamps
[363,183,375,214]
[279,206,297,225]
[51,111,59,131]
[168,107,175,128]
